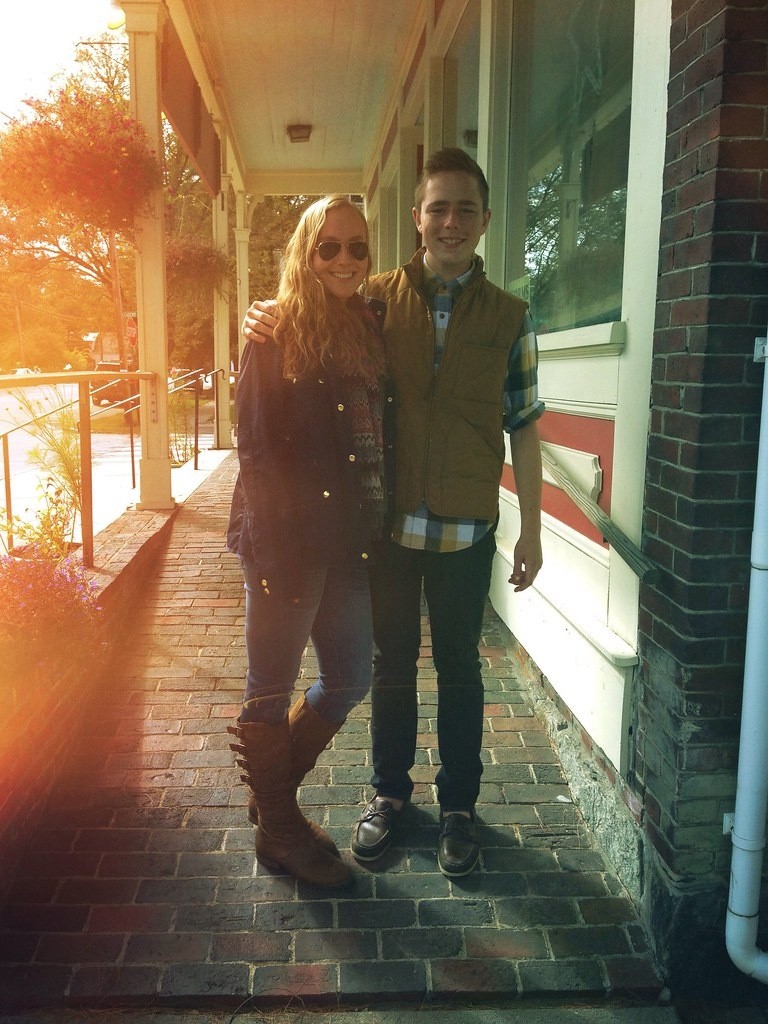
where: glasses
[314,241,369,261]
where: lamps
[287,124,312,143]
[463,130,478,147]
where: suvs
[90,358,137,406]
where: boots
[226,711,352,890]
[247,686,337,855]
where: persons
[240,147,543,877]
[227,194,387,884]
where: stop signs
[126,318,139,345]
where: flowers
[168,374,198,464]
[0,382,79,559]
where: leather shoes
[350,792,411,861]
[440,804,480,877]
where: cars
[5,368,35,375]
[136,367,203,393]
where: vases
[172,464,181,468]
[8,541,82,562]
